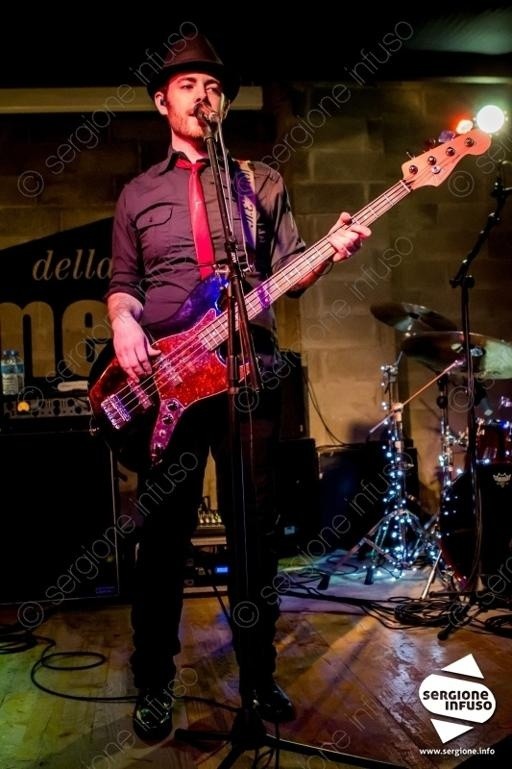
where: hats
[145,34,242,103]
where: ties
[173,156,216,280]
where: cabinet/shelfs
[0,395,126,611]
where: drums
[438,470,511,606]
[476,421,511,466]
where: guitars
[88,128,492,474]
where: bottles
[1,348,26,394]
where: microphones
[192,101,219,125]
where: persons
[104,32,372,740]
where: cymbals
[370,300,458,335]
[401,331,511,380]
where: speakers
[246,440,317,564]
[253,352,307,437]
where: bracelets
[311,261,335,277]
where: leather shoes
[130,671,179,745]
[237,667,296,723]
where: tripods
[317,363,462,604]
[171,122,404,768]
